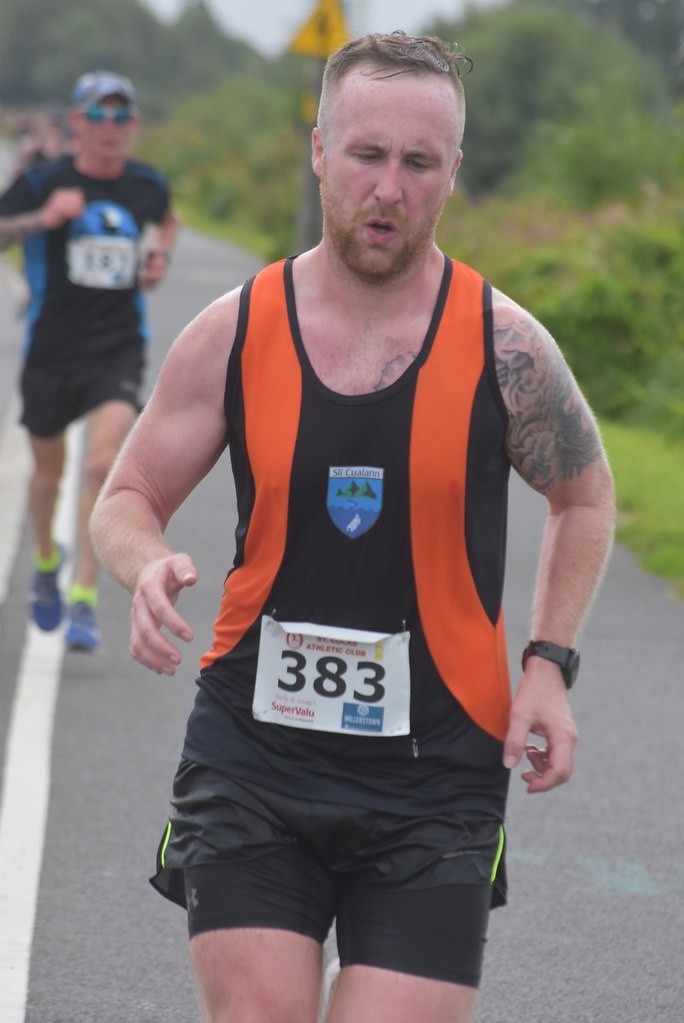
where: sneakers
[31,542,62,634]
[68,585,98,652]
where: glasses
[77,105,133,126]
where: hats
[73,70,133,113]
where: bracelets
[148,248,171,261]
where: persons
[89,28,617,1023]
[0,68,178,653]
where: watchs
[522,639,581,690]
